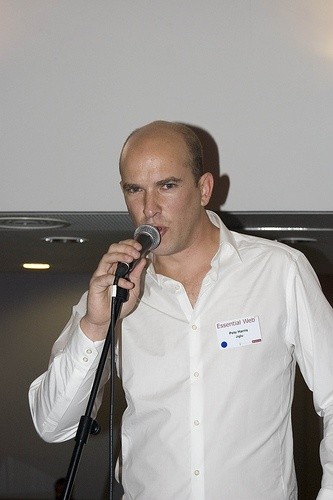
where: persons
[27,118,333,500]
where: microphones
[114,224,161,278]
